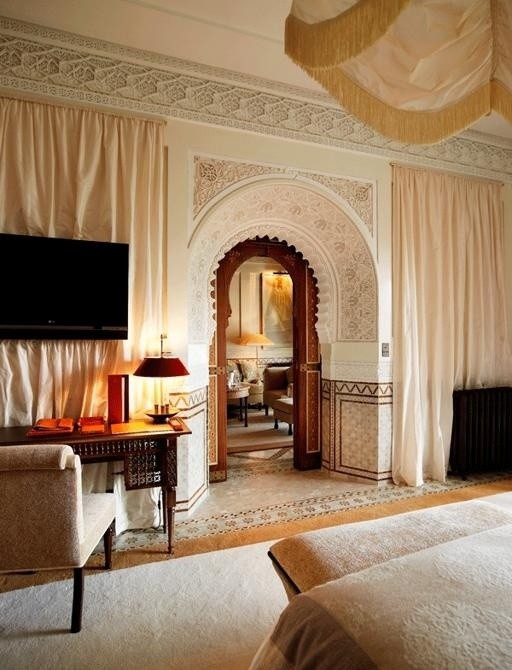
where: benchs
[268,500,511,602]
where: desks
[0,416,192,554]
[227,383,251,427]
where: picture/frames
[260,272,294,348]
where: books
[26,418,75,436]
[78,416,106,433]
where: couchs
[227,358,262,411]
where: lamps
[133,333,191,424]
[240,334,274,381]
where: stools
[274,398,294,436]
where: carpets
[1,490,511,669]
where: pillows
[239,360,259,383]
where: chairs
[0,444,117,634]
[262,366,294,417]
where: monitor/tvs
[0,232,129,340]
